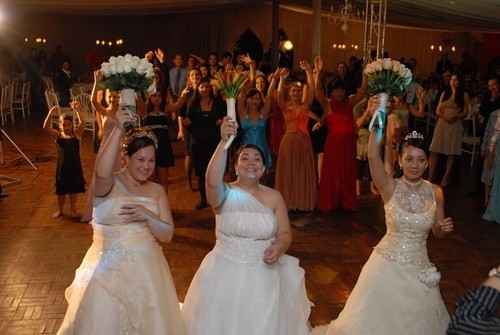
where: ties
[174,69,180,95]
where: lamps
[327,0,364,34]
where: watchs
[488,268,500,278]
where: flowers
[96,53,155,126]
[209,68,249,98]
[365,58,414,131]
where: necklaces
[122,168,137,187]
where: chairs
[40,77,96,141]
[424,92,436,134]
[0,72,33,127]
[461,115,481,167]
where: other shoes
[195,202,207,210]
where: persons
[58,103,186,335]
[179,115,314,335]
[326,96,454,335]
[446,263,500,335]
[43,48,500,223]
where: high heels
[440,179,449,187]
[428,176,437,182]
